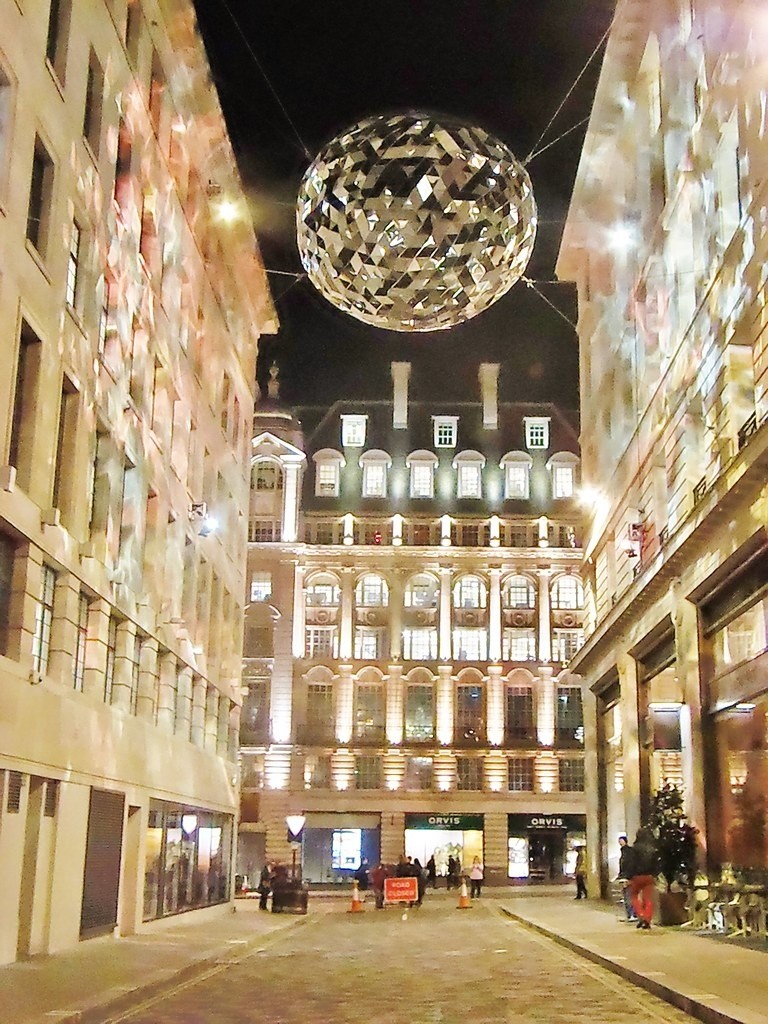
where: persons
[445,854,457,891]
[258,858,278,912]
[234,874,242,895]
[572,843,590,901]
[402,854,416,877]
[615,835,641,924]
[395,853,406,878]
[463,726,481,746]
[454,856,463,888]
[468,854,486,903]
[370,861,394,909]
[425,855,439,890]
[352,856,372,903]
[567,531,581,547]
[410,858,427,908]
[432,841,463,877]
[372,529,382,545]
[264,593,270,600]
[628,827,661,929]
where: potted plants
[635,787,701,931]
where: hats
[620,836,627,843]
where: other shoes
[470,898,475,901]
[476,898,479,901]
[585,890,587,898]
[628,915,638,922]
[643,923,650,928]
[637,920,646,928]
[575,896,581,899]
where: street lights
[285,813,306,881]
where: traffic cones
[346,879,365,913]
[455,876,474,910]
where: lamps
[188,499,214,539]
